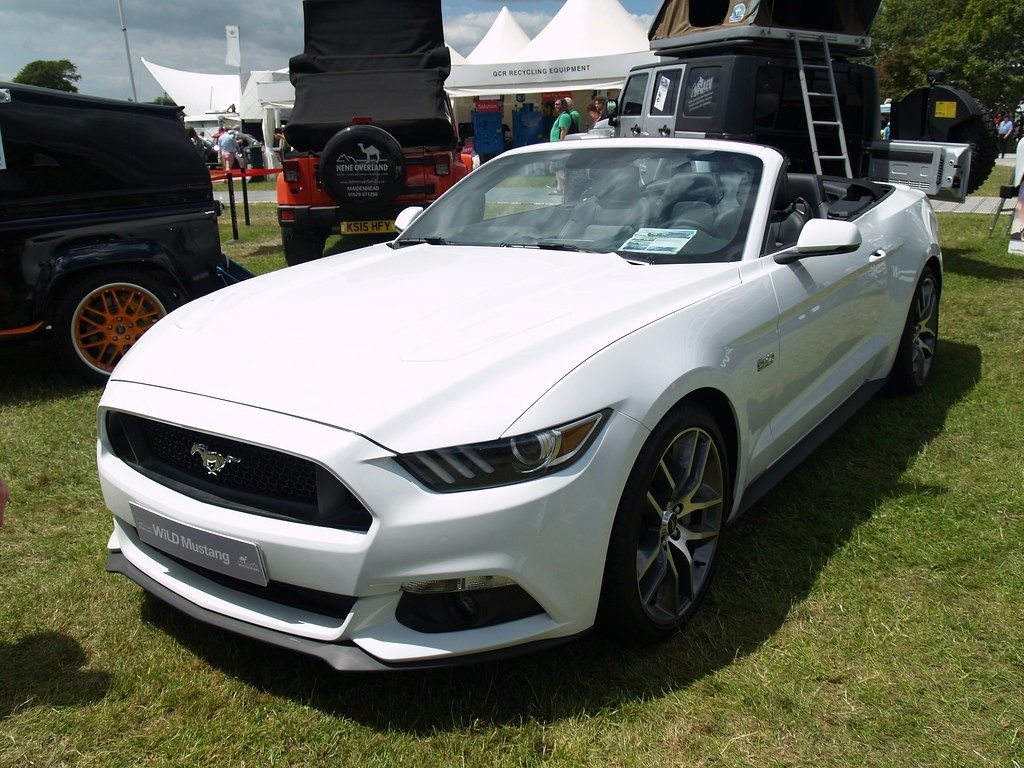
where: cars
[93,135,944,677]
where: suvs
[0,80,229,388]
[548,1,1001,209]
[274,0,480,266]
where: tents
[443,0,677,98]
[257,80,295,180]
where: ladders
[987,169,1021,238]
[791,32,853,179]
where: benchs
[658,170,831,223]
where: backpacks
[558,113,580,139]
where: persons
[185,124,244,171]
[550,98,571,195]
[883,122,891,140]
[585,96,607,122]
[994,113,1024,240]
[548,97,582,187]
[273,128,288,152]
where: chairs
[561,162,648,249]
[712,168,803,250]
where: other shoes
[548,188,564,195]
